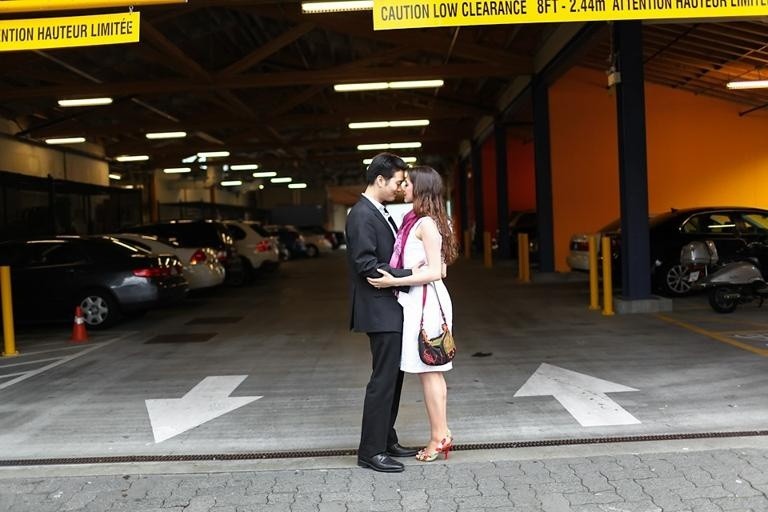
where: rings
[377,286,380,289]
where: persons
[365,164,461,462]
[344,151,448,474]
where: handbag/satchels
[419,324,457,365]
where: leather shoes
[387,442,420,457]
[358,453,405,472]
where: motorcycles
[679,235,767,314]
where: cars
[567,213,657,275]
[597,206,767,297]
[488,209,539,263]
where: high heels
[416,428,453,461]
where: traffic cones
[67,304,92,344]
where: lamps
[333,79,444,171]
[46,97,308,191]
[725,80,768,91]
[301,0,373,15]
